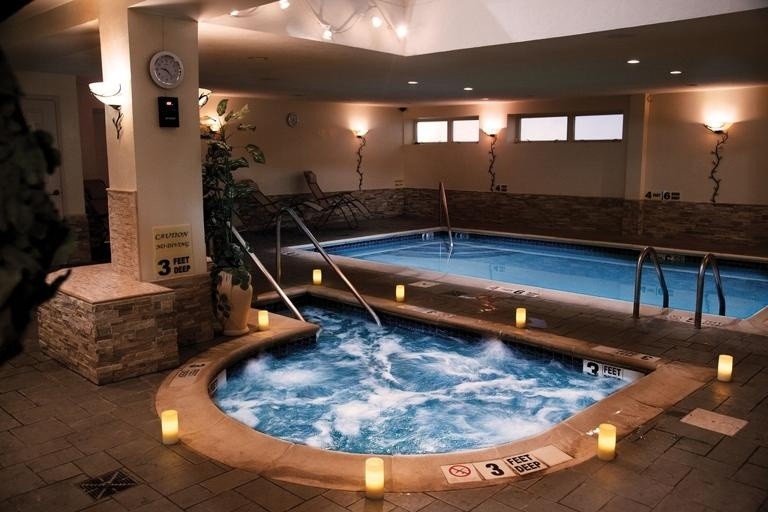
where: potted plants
[199,96,266,337]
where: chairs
[242,170,373,237]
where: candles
[312,269,322,285]
[515,307,527,329]
[716,354,734,383]
[395,284,406,303]
[257,310,269,331]
[364,457,385,500]
[597,423,617,462]
[161,407,180,446]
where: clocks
[149,51,185,89]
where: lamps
[481,118,502,192]
[352,124,368,190]
[88,81,129,139]
[703,111,735,202]
